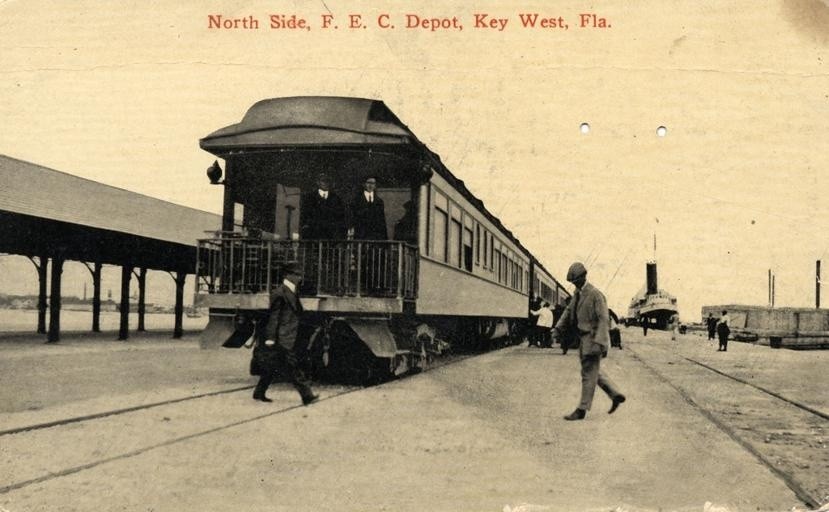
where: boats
[626,289,679,330]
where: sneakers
[304,395,319,405]
[253,392,272,402]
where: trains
[192,96,572,386]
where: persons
[557,294,573,355]
[641,313,650,336]
[551,262,626,420]
[552,303,563,343]
[704,312,717,340]
[669,310,680,342]
[298,168,350,297]
[529,301,553,348]
[347,174,389,297]
[527,296,543,347]
[255,261,320,405]
[716,310,730,351]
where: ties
[573,292,579,332]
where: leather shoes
[609,394,625,414]
[564,408,585,420]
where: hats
[567,262,587,283]
[286,261,305,276]
[318,171,333,181]
